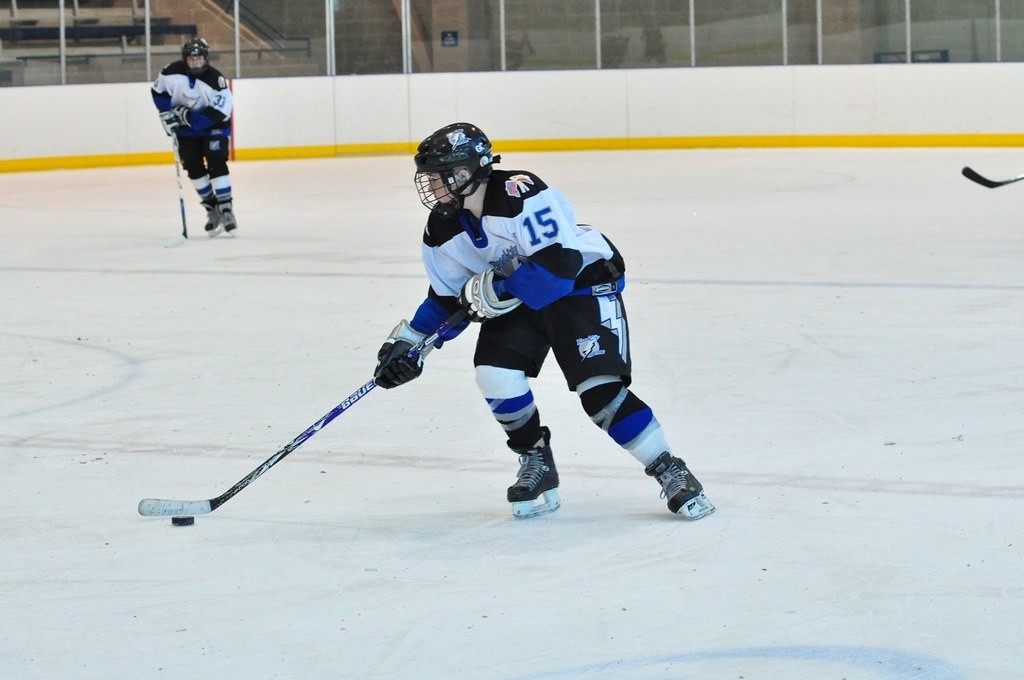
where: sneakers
[217,197,237,238]
[507,426,561,519]
[645,452,716,520]
[200,196,223,236]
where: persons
[149,37,238,240]
[373,123,716,521]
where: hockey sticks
[137,304,471,518]
[161,131,189,249]
[962,166,1024,189]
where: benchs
[0,0,221,83]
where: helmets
[181,37,210,65]
[414,123,493,179]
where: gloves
[160,111,180,136]
[448,268,523,324]
[373,318,435,389]
[173,105,191,128]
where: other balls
[170,515,195,526]
